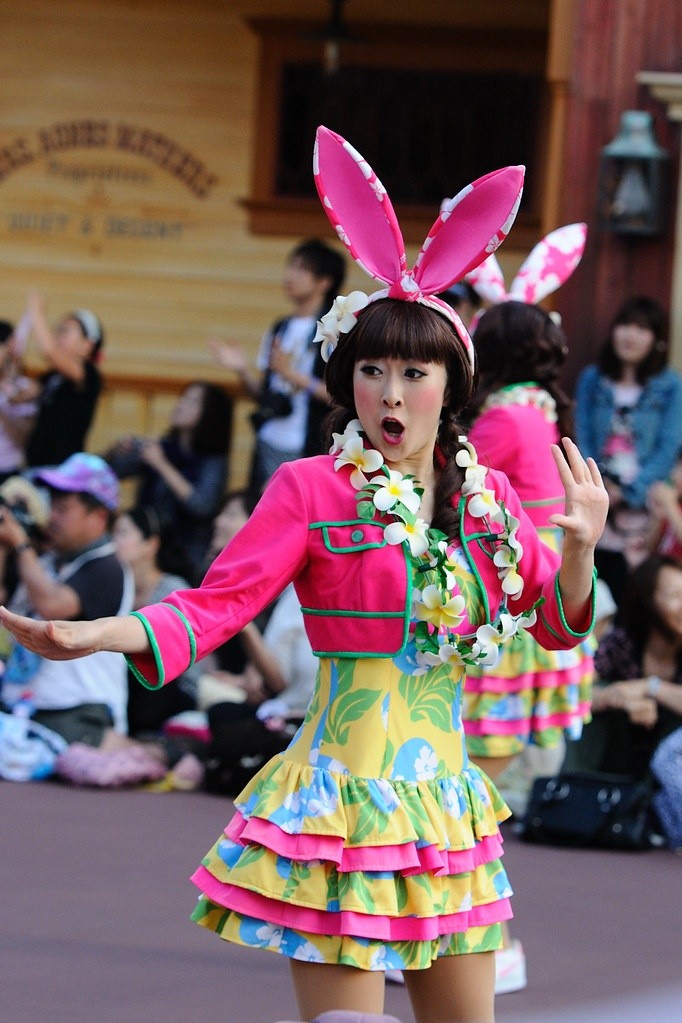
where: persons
[0,298,318,772]
[0,297,611,1023]
[213,237,348,483]
[454,300,598,782]
[590,448,682,783]
[574,297,682,596]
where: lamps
[602,87,668,234]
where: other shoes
[488,934,532,998]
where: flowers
[312,291,368,363]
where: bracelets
[14,543,32,555]
[646,675,659,698]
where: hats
[29,451,121,514]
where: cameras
[249,392,292,431]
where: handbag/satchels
[518,764,661,853]
